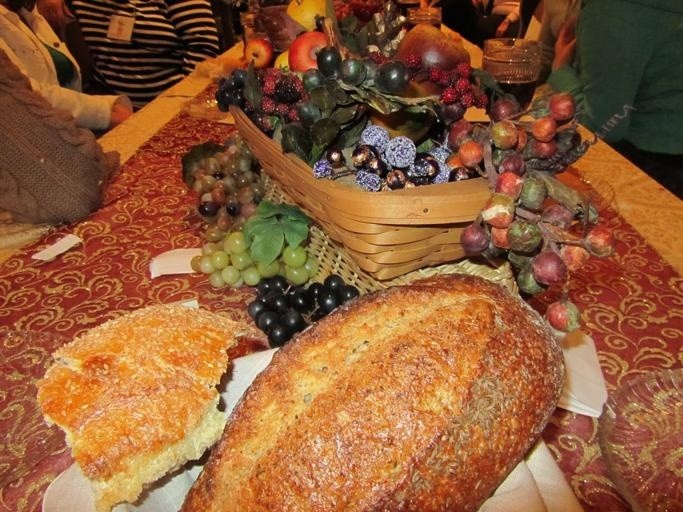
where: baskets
[227,101,495,284]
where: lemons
[274,51,289,68]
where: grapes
[215,68,246,112]
[189,140,358,347]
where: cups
[238,12,268,57]
[407,6,443,32]
[483,38,542,111]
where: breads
[180,273,564,511]
[38,302,255,512]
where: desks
[1,22,683,512]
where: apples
[286,0,383,31]
[244,38,273,67]
[288,31,327,71]
[393,24,471,71]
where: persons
[494,0,581,86]
[0,1,135,132]
[0,48,111,229]
[36,0,220,114]
[545,0,682,200]
